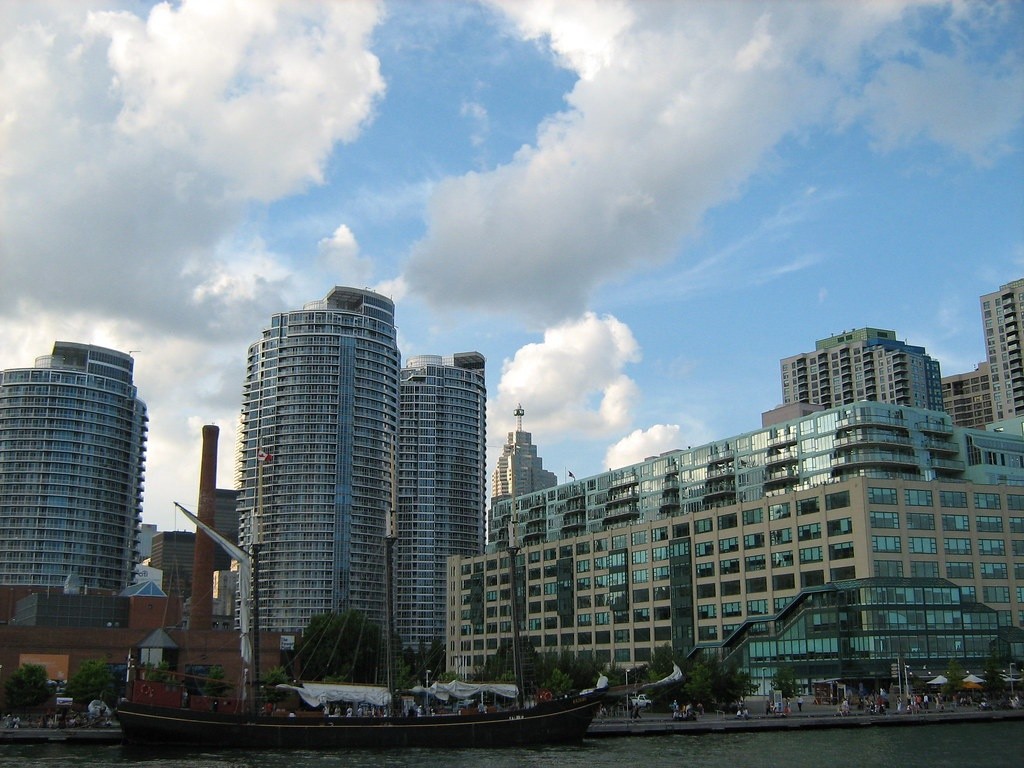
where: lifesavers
[544,692,553,702]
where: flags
[569,472,575,480]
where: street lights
[623,667,631,727]
[904,665,910,698]
[425,670,432,715]
[1010,663,1017,695]
[762,666,767,714]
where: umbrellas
[926,675,987,695]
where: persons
[629,699,641,720]
[837,687,1021,716]
[601,703,607,724]
[182,689,488,716]
[670,700,704,721]
[5,712,113,729]
[737,700,748,720]
[766,696,803,717]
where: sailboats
[116,499,688,751]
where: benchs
[977,705,992,711]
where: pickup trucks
[628,694,652,711]
